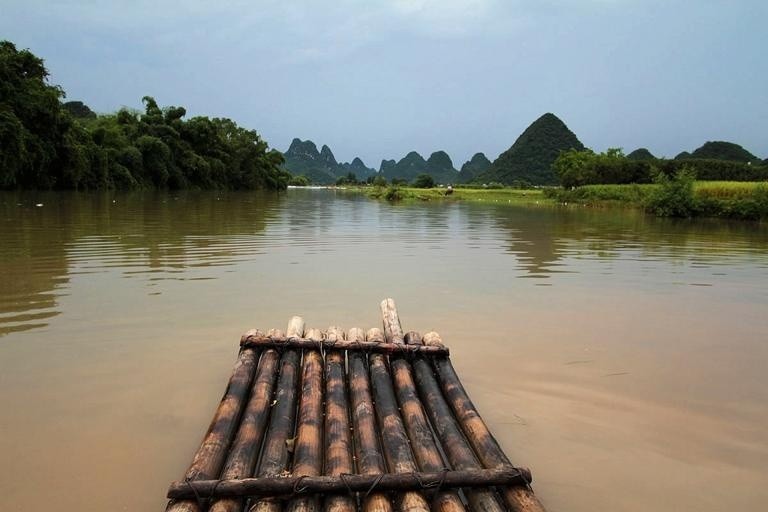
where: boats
[161,296,545,511]
